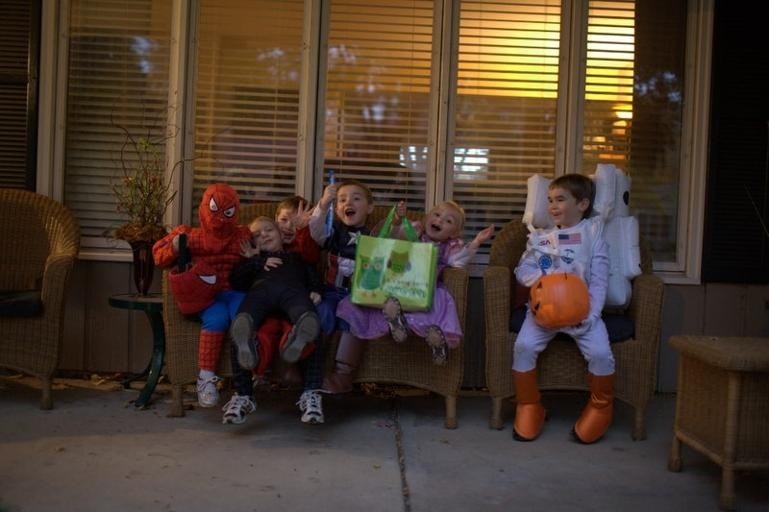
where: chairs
[480,216,669,444]
[0,185,84,412]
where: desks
[107,292,166,412]
[662,329,768,509]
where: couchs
[160,197,472,430]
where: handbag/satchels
[351,202,439,312]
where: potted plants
[102,75,232,299]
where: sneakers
[383,296,408,344]
[424,324,449,368]
[298,393,324,424]
[281,312,322,365]
[231,313,261,371]
[196,377,222,410]
[222,393,257,426]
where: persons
[149,182,316,409]
[231,213,321,372]
[513,173,612,446]
[221,195,328,427]
[316,198,496,395]
[295,179,380,414]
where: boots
[574,373,613,447]
[321,330,362,394]
[511,367,546,440]
